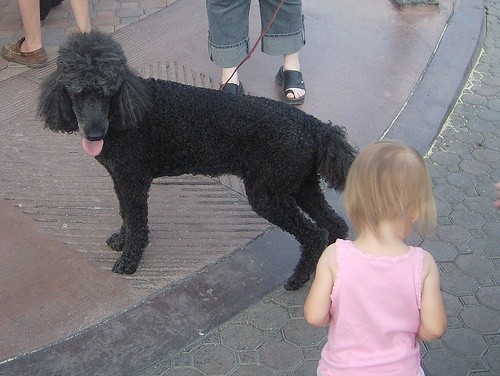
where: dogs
[34,27,360,291]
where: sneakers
[0,36,47,67]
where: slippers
[275,66,306,105]
[219,81,245,96]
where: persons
[204,0,307,106]
[0,0,103,71]
[303,141,448,376]
